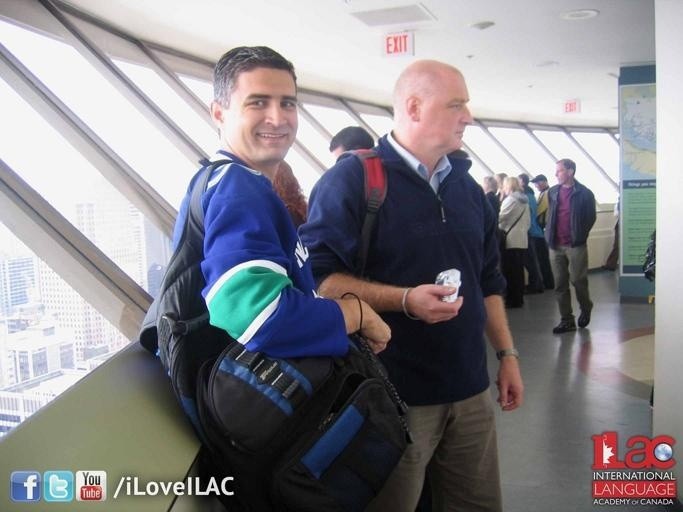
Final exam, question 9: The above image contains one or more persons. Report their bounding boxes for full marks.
[293,59,528,512]
[137,46,393,512]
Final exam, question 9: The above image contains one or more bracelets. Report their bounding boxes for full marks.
[497,347,521,361]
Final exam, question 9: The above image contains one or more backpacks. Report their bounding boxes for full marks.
[138,159,413,511]
[643,231,655,281]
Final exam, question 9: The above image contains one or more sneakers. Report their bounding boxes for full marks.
[553,322,576,333]
[578,302,593,327]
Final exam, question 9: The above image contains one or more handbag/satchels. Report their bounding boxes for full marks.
[497,230,506,250]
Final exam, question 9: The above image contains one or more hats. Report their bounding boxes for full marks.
[529,174,546,182]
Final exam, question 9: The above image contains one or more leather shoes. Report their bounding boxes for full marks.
[524,285,554,294]
[604,266,616,271]
[504,295,524,308]
